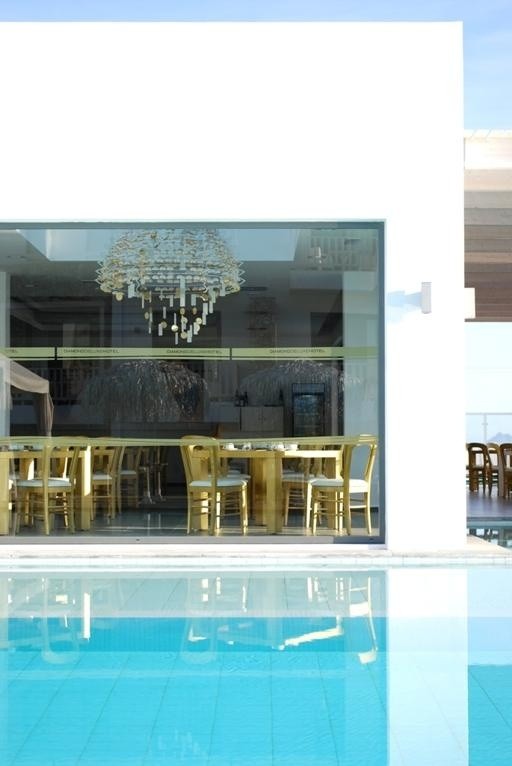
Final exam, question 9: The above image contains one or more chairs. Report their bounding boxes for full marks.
[188,577,378,666]
[466,442,512,499]
[119,446,170,510]
[180,434,378,537]
[0,435,126,536]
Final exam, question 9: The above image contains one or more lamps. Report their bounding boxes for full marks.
[93,229,246,346]
